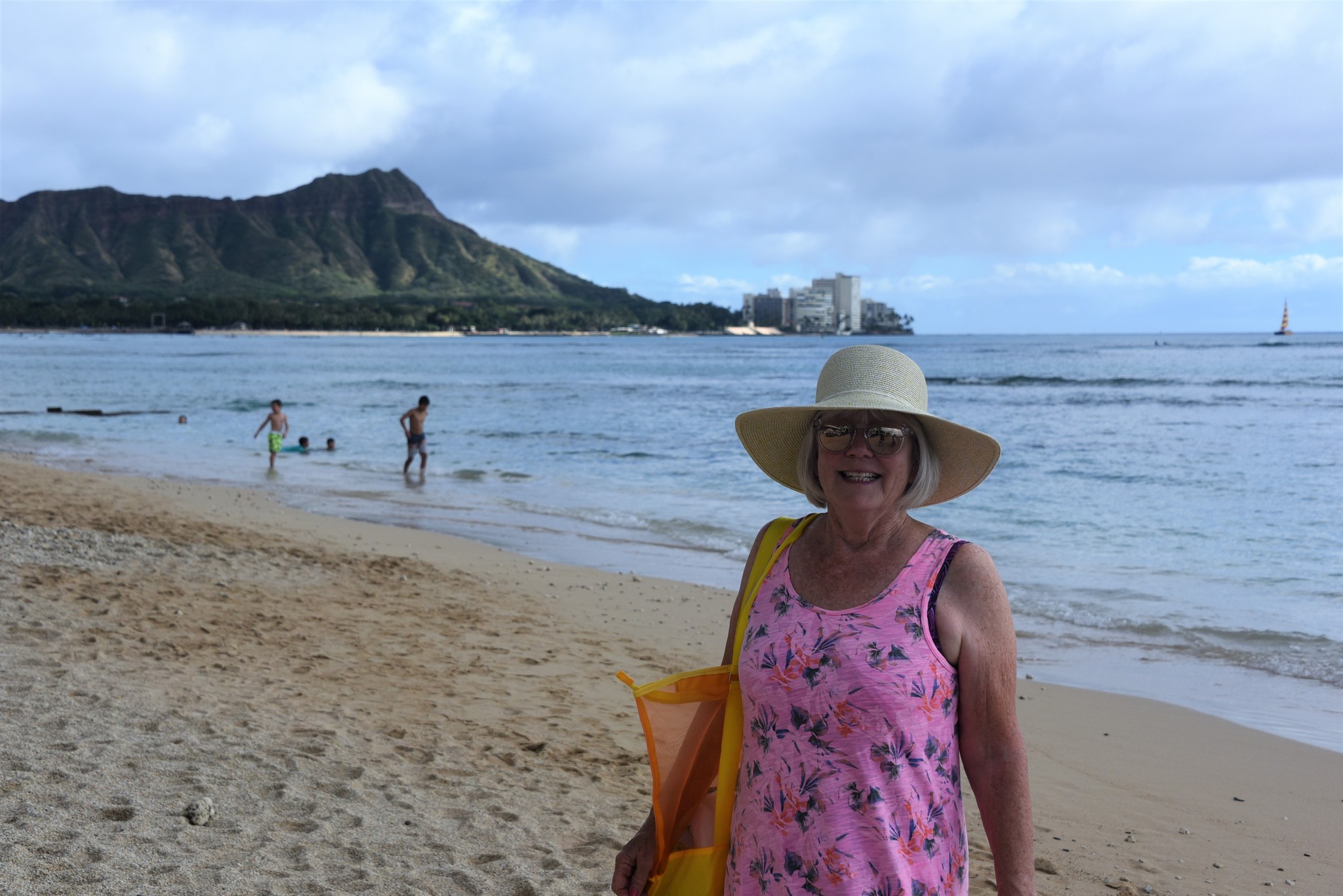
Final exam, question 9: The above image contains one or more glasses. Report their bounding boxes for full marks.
[814,421,918,458]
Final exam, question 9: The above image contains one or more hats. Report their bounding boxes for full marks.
[734,344,1001,509]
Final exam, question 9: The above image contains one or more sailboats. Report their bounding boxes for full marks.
[1274,298,1293,336]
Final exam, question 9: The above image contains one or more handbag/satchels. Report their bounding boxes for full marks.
[616,518,795,896]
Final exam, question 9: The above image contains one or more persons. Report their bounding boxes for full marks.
[253,399,289,468]
[403,473,426,495]
[325,437,337,451]
[298,436,310,453]
[609,344,1039,896]
[398,394,432,471]
[178,414,188,424]
